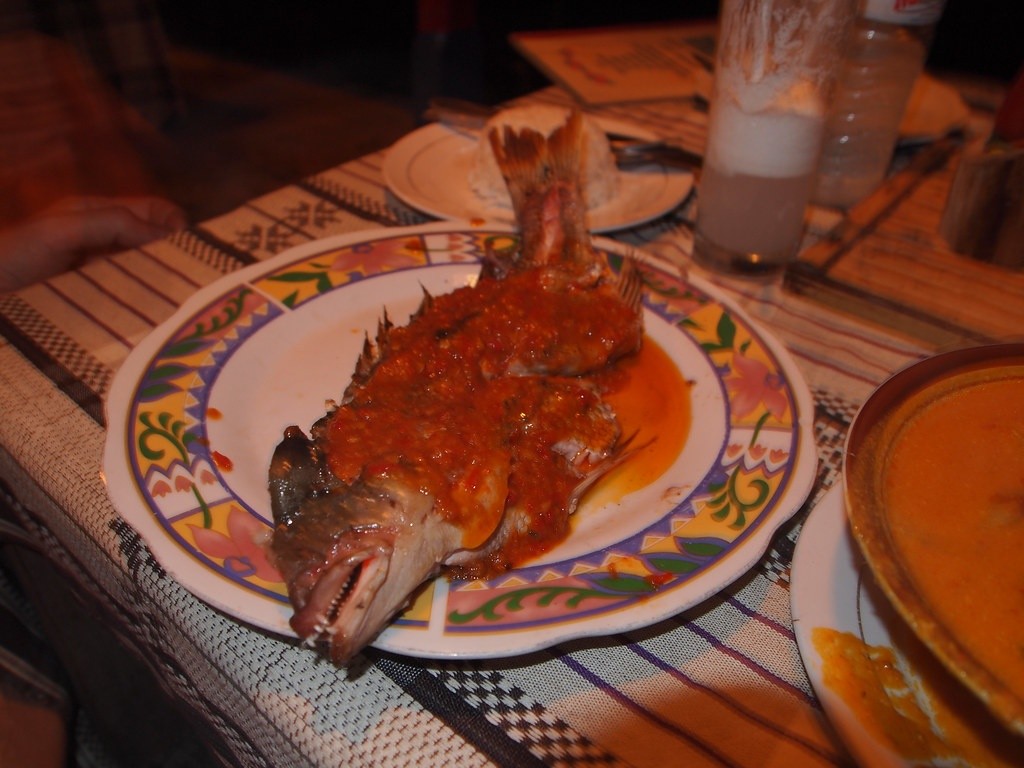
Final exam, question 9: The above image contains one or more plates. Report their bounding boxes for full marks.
[776,70,963,146]
[106,222,818,660]
[385,120,695,235]
[791,479,1024,768]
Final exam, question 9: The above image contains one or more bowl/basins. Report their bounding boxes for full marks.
[842,342,1024,743]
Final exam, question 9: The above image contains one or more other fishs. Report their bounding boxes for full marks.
[267,102,645,668]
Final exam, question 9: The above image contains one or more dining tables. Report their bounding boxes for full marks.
[0,85,1024,766]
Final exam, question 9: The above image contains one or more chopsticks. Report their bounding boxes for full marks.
[800,123,970,272]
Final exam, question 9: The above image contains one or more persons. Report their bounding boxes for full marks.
[0,193,191,768]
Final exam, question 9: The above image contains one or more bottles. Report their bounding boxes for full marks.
[804,0,941,237]
[694,0,850,286]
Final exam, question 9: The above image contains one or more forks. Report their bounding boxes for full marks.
[422,104,682,168]
